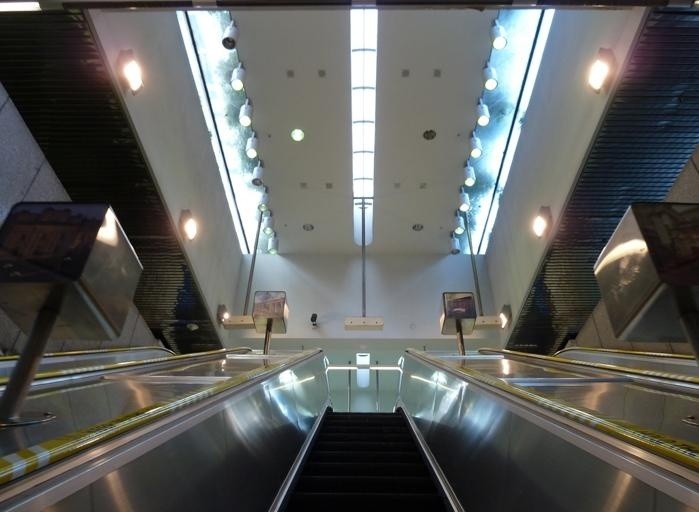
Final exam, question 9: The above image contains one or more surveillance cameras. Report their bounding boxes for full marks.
[310,313,318,326]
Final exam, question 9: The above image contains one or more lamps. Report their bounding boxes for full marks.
[110,47,147,94]
[527,206,553,239]
[492,305,514,328]
[220,18,278,256]
[450,19,507,255]
[583,47,617,92]
[176,208,198,245]
[217,304,232,327]
